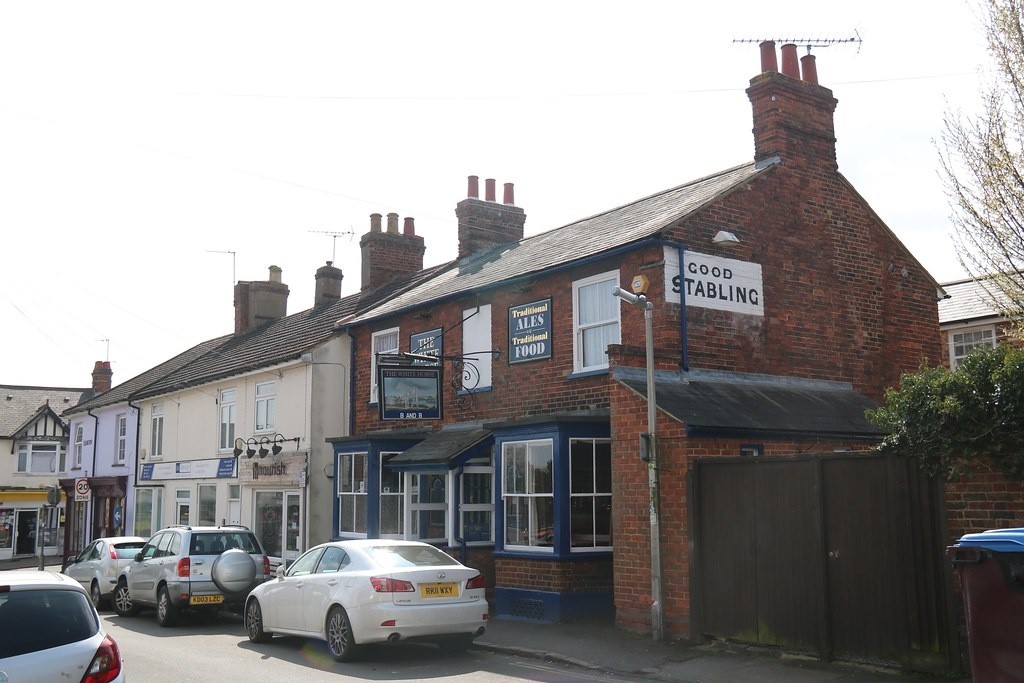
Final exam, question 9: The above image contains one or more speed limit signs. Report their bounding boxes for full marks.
[76,478,90,494]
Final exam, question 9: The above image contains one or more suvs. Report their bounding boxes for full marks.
[111,524,271,628]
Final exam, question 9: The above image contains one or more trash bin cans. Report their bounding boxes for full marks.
[946,528,1023,682]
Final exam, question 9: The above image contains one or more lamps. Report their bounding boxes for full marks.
[233,434,300,459]
[711,231,741,247]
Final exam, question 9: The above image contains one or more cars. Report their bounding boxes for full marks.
[64,536,152,610]
[244,539,489,662]
[0,570,126,683]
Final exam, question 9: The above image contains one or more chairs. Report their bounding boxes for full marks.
[194,540,224,552]
